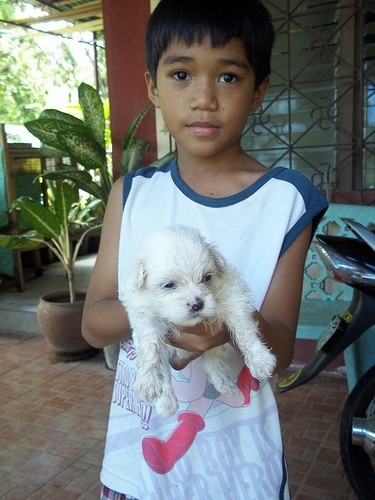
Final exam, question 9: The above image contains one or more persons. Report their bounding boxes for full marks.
[80,0,330,500]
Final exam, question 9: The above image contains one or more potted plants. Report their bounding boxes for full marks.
[0,81,177,362]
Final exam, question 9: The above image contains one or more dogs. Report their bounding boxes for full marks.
[118,224,278,418]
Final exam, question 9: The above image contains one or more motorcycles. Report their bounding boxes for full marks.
[274,216,375,500]
